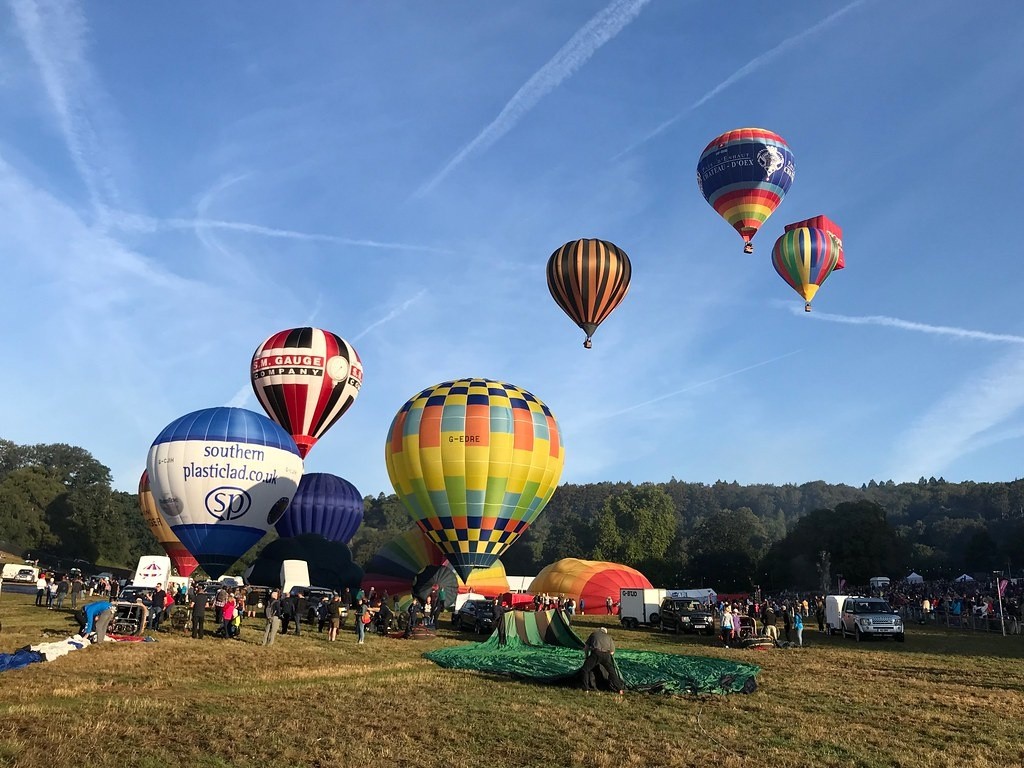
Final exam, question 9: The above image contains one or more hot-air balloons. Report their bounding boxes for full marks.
[138,468,200,576]
[771,227,840,313]
[360,527,509,621]
[695,127,798,254]
[384,377,567,599]
[146,406,304,583]
[274,472,365,547]
[524,558,654,616]
[250,327,364,463]
[784,215,845,270]
[245,532,364,591]
[545,238,633,350]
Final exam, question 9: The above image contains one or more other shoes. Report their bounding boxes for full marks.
[723,644,729,648]
[355,640,364,645]
[47,607,53,610]
[71,606,77,611]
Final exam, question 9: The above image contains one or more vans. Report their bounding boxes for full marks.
[288,586,348,626]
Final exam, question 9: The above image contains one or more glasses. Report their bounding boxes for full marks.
[156,586,159,588]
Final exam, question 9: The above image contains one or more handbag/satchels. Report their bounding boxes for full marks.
[361,611,371,623]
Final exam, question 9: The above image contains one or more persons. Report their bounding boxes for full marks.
[532,593,622,616]
[664,571,1024,648]
[36,569,446,648]
[579,626,626,694]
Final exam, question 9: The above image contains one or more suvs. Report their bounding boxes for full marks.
[841,595,906,642]
[660,596,715,637]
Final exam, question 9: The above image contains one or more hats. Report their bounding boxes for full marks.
[50,578,54,581]
[39,574,46,578]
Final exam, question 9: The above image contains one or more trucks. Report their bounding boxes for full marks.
[618,587,669,630]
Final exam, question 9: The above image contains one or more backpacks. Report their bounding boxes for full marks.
[216,590,226,602]
[265,600,280,622]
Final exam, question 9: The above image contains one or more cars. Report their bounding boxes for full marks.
[110,585,158,628]
[456,597,497,637]
[14,569,35,584]
